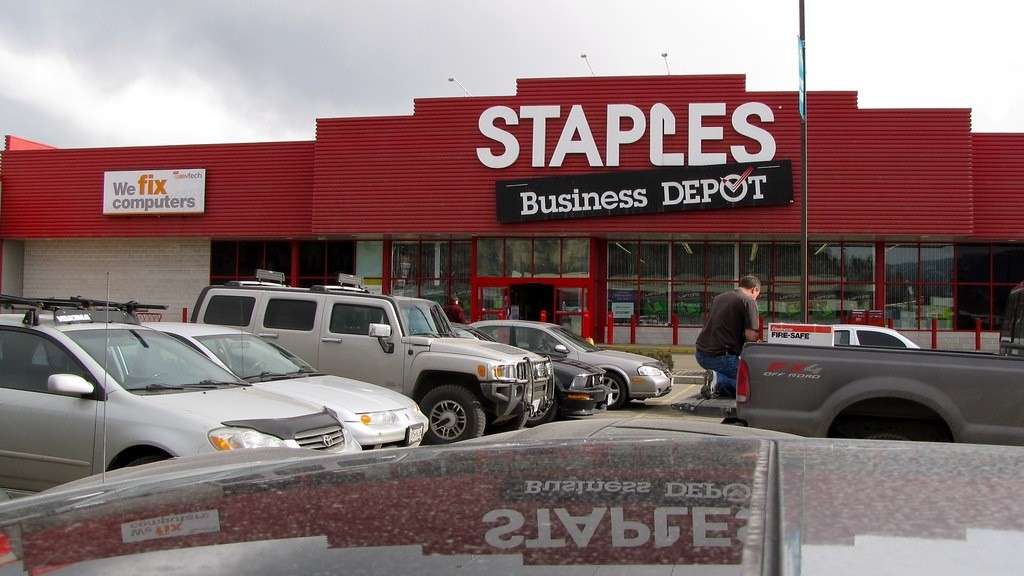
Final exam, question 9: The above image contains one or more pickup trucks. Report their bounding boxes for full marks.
[719,343,1024,447]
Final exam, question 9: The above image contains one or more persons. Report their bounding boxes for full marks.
[695,276,761,400]
[443,296,466,325]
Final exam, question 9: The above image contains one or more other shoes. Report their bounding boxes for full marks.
[701,369,717,399]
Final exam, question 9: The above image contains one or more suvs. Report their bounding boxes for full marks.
[188,270,554,445]
[1,292,365,503]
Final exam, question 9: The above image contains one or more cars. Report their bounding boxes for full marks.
[640,316,662,325]
[828,324,927,350]
[450,322,609,428]
[114,320,429,449]
[469,319,675,411]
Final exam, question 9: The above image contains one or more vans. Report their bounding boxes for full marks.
[997,281,1024,357]
